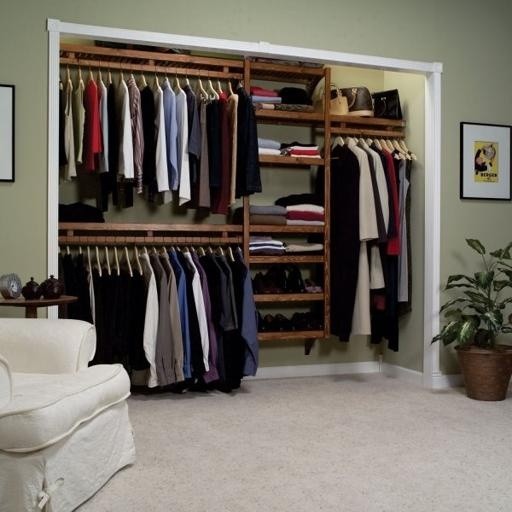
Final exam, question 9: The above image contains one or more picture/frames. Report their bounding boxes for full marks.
[460,122,512,201]
[0,83,16,183]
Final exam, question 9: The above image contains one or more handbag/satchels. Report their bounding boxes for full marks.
[315,83,403,120]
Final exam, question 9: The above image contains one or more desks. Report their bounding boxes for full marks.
[0,296,78,320]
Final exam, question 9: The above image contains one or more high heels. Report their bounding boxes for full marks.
[253,264,323,293]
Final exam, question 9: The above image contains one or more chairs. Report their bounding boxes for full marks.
[0,316,136,512]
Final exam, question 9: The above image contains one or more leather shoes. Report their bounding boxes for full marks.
[255,311,324,332]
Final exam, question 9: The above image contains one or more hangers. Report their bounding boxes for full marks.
[60,60,241,103]
[332,125,416,166]
[62,231,243,277]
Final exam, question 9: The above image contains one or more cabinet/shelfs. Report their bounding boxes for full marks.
[59,44,407,343]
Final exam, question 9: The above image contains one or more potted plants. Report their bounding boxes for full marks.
[427,239,512,404]
